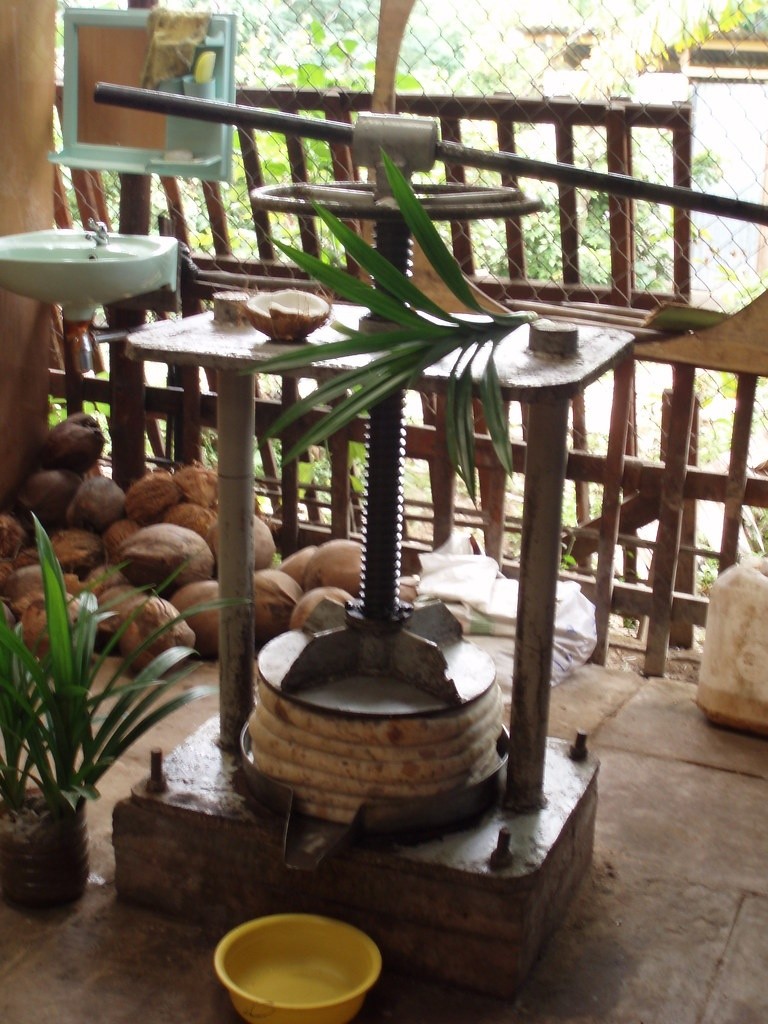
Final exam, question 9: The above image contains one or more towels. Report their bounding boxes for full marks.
[142,6,212,90]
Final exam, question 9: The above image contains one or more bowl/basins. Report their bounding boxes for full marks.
[213,914,382,1024]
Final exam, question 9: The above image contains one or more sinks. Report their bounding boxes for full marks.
[0,228,179,305]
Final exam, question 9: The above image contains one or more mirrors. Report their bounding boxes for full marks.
[74,25,163,150]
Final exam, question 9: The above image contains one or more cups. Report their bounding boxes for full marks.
[183,76,215,99]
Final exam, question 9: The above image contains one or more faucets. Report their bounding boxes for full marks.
[85,217,109,246]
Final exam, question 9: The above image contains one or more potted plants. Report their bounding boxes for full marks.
[1,504,256,908]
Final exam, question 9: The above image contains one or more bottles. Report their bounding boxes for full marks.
[694,556,768,734]
